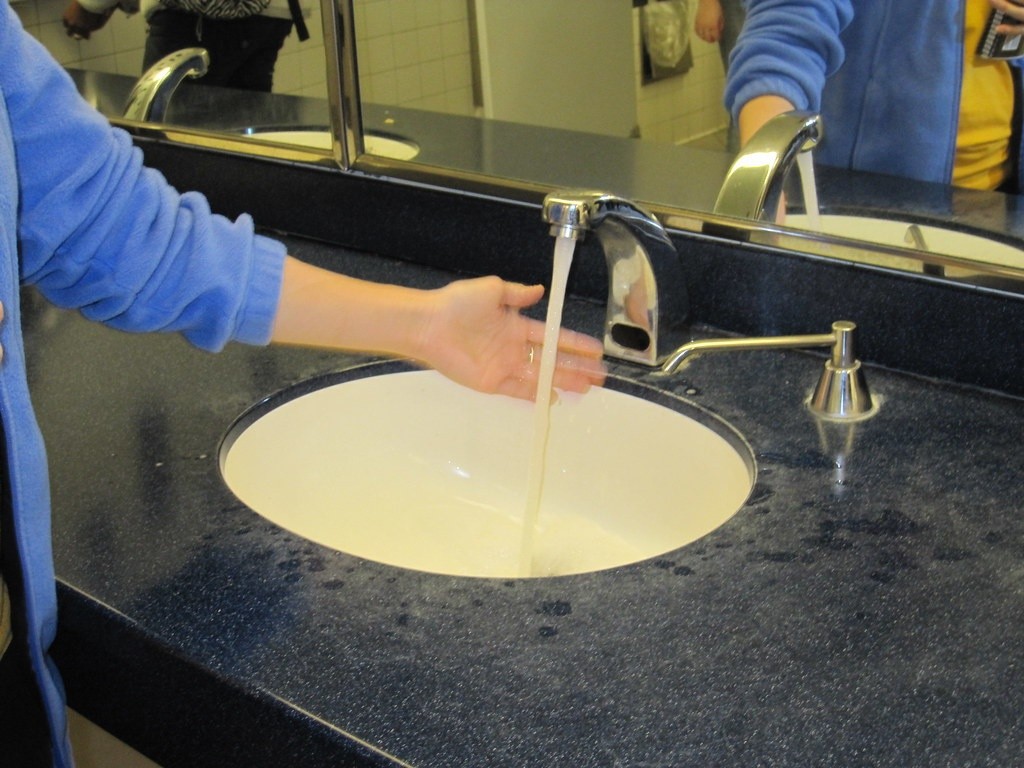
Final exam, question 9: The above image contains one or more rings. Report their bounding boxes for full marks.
[524,344,534,363]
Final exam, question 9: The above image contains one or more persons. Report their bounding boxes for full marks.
[724,0,1024,188]
[693,0,753,72]
[0,0,608,768]
[62,0,304,95]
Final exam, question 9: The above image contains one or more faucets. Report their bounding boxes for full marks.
[709,104,827,231]
[121,41,210,121]
[537,182,697,371]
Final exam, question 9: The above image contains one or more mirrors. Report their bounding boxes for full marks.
[10,0,1024,278]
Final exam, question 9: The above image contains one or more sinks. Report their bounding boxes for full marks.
[214,349,761,587]
[663,200,1024,292]
[232,116,421,171]
